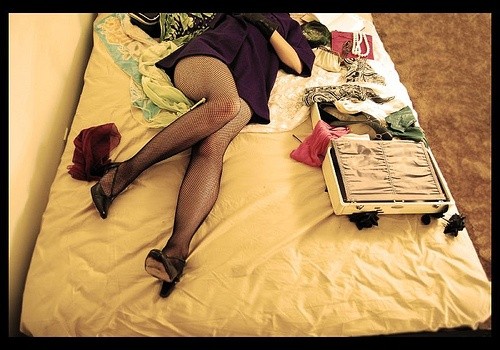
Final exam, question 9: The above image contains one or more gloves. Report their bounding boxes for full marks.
[231,13,279,40]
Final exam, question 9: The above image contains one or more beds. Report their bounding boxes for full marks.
[20,12,491,337]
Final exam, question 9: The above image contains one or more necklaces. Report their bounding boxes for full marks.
[352,33,361,55]
[355,33,370,57]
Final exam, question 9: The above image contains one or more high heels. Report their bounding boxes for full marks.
[144,249,185,298]
[91,161,118,219]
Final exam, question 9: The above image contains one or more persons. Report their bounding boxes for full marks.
[89,13,315,297]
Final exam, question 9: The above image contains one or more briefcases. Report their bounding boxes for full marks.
[310,102,455,215]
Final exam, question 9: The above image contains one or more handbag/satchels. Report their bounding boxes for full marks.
[332,30,374,59]
